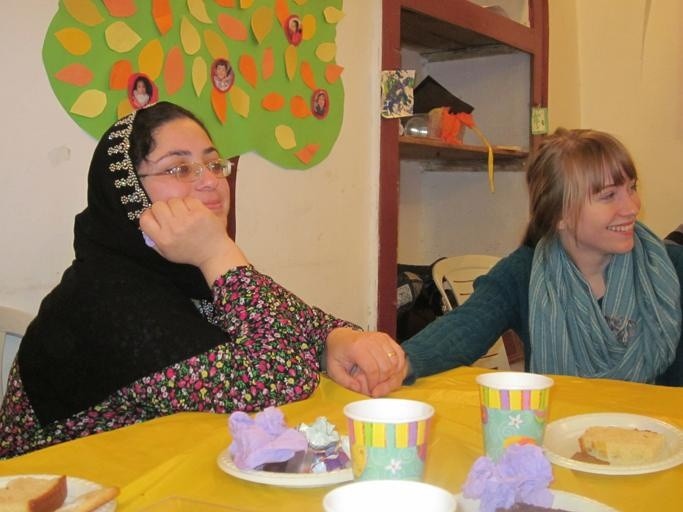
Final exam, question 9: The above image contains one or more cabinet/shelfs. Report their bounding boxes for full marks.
[377,0,549,341]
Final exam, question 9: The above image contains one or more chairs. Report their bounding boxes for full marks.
[432,254,511,371]
[0,305,36,408]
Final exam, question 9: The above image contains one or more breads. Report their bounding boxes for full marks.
[570,427,667,466]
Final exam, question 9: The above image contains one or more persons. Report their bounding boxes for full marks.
[1,101,407,462]
[288,19,302,46]
[348,121,683,399]
[131,79,150,107]
[211,59,232,92]
[312,91,325,115]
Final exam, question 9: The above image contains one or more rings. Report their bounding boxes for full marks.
[387,352,397,357]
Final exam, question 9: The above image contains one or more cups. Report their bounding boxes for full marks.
[342,398,436,489]
[475,370,555,463]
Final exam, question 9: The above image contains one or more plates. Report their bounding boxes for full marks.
[322,478,459,511]
[214,435,353,488]
[452,487,620,511]
[545,411,681,478]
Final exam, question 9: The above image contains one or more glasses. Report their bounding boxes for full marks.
[138,159,234,183]
